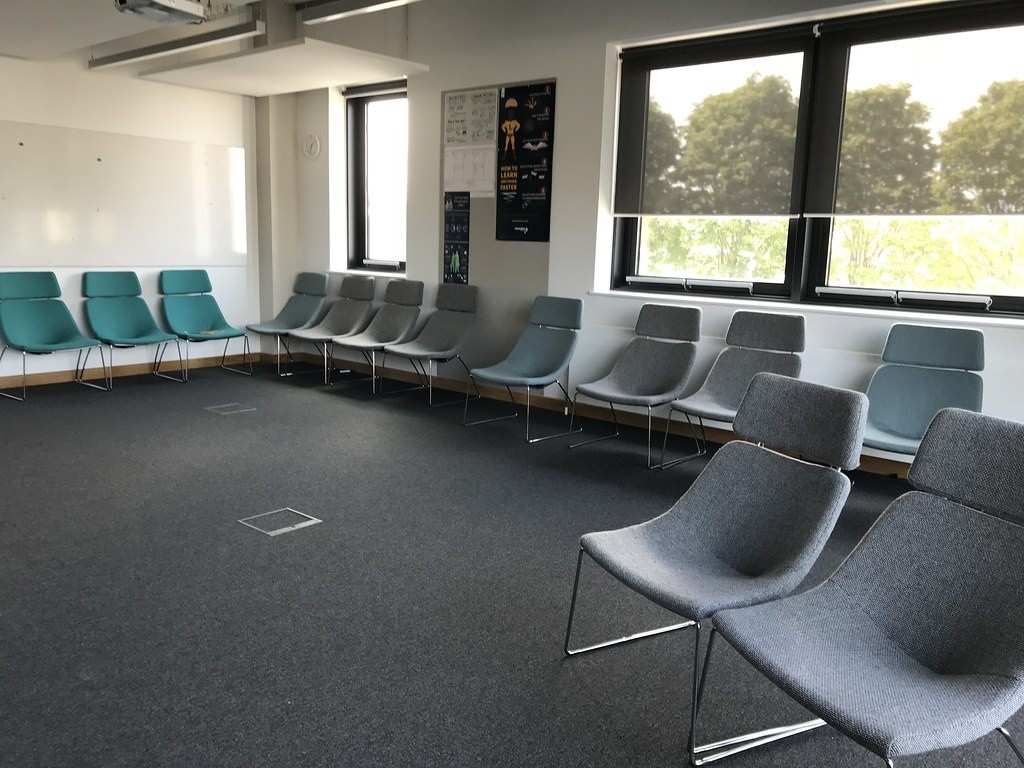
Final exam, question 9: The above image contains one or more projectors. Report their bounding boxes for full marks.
[113,0,204,26]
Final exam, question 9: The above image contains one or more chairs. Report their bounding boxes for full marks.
[243,272,328,377]
[837,324,984,487]
[566,304,701,469]
[155,269,254,382]
[74,272,186,392]
[0,271,112,403]
[376,284,483,407]
[661,312,805,466]
[689,407,1024,768]
[287,275,376,381]
[326,278,424,400]
[466,297,587,443]
[565,372,869,748]
